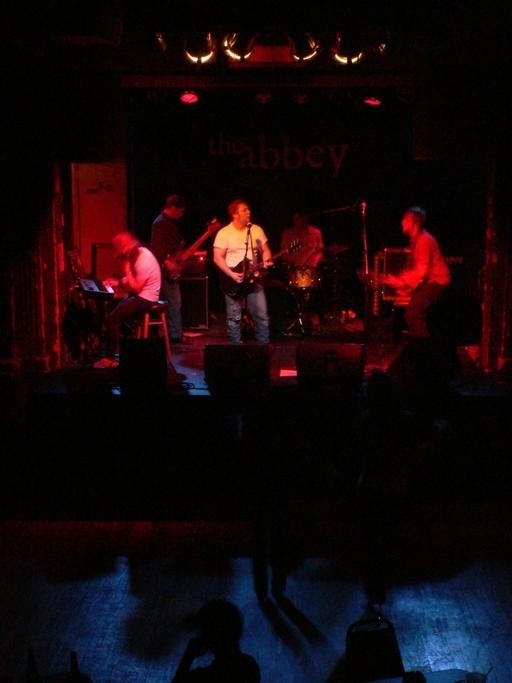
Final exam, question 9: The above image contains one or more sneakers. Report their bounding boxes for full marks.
[172,335,192,345]
[93,359,118,367]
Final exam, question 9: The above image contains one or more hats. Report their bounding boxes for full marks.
[111,233,137,258]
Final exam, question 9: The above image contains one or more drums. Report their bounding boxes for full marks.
[285,262,321,291]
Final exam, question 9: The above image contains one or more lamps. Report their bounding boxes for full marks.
[178,30,393,111]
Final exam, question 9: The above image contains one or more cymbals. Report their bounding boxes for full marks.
[319,244,349,254]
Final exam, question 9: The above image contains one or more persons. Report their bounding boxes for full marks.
[174,598,262,683]
[106,229,162,353]
[276,210,326,324]
[214,200,275,344]
[381,205,451,336]
[149,193,188,341]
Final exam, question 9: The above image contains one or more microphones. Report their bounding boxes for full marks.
[244,222,254,232]
[360,198,367,217]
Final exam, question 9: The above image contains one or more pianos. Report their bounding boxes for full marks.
[80,279,115,299]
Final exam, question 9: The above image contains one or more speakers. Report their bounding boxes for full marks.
[386,336,459,385]
[118,335,168,384]
[204,344,270,388]
[177,274,209,330]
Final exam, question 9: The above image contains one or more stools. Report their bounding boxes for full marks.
[135,295,176,375]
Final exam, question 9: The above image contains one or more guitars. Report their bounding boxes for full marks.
[222,240,305,300]
[162,218,222,283]
